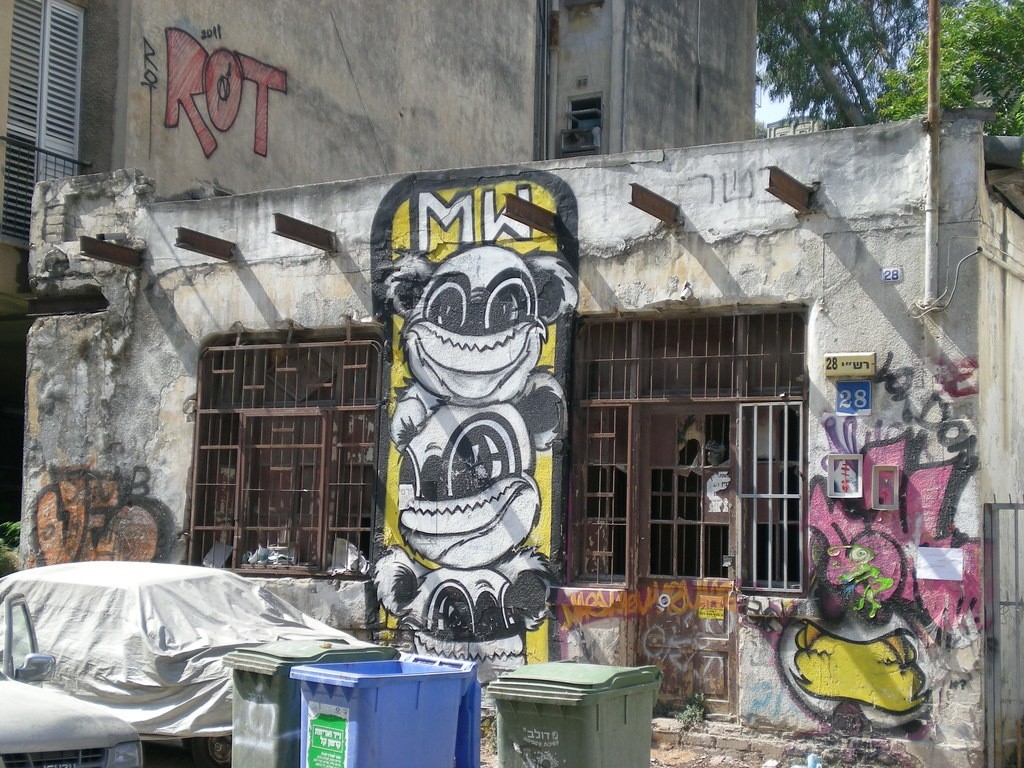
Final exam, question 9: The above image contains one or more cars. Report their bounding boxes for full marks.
[0,592,142,768]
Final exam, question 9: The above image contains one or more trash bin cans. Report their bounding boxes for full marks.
[485,659,664,768]
[222,635,401,768]
[287,652,480,768]
